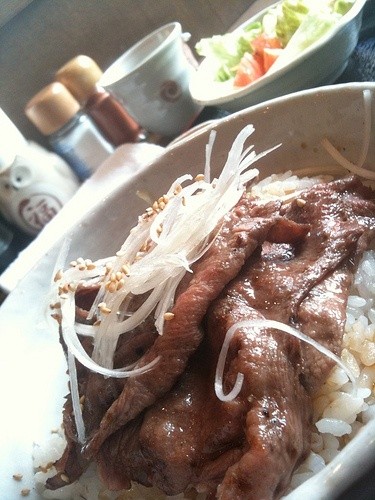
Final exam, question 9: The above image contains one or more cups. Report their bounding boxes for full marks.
[98,21,204,141]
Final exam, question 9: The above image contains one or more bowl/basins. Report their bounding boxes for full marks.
[0,80,375,499]
[189,1,367,114]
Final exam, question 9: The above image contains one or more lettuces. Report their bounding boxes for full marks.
[194,0,362,90]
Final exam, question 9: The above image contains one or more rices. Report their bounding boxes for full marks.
[32,169,374,499]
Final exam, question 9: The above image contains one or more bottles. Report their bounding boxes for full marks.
[24,81,114,180]
[0,108,82,237]
[50,55,142,148]
